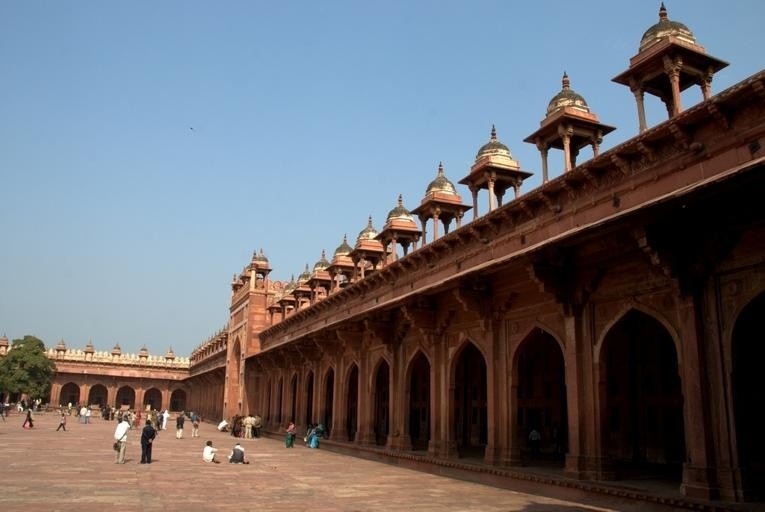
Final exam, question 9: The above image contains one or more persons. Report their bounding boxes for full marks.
[203,441,221,464]
[285,420,297,449]
[304,422,327,448]
[138,420,157,464]
[56,413,66,432]
[113,415,131,464]
[228,443,245,464]
[99,400,170,431]
[176,410,185,439]
[529,428,540,441]
[0,397,42,428]
[218,413,263,439]
[191,412,200,437]
[59,399,91,424]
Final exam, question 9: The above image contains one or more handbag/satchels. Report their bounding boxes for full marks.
[113,443,120,452]
